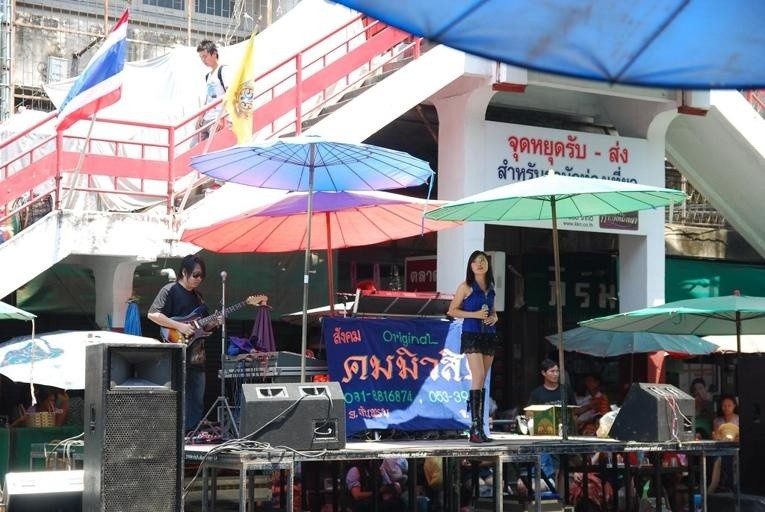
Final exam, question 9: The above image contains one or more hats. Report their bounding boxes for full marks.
[719,422,740,441]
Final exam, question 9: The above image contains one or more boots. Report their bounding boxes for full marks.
[466,389,484,443]
[480,388,493,442]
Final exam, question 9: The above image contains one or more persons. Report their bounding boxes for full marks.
[194,40,231,145]
[581,367,607,418]
[707,398,738,496]
[529,360,578,406]
[447,250,499,443]
[691,380,714,418]
[147,253,222,438]
[267,445,705,512]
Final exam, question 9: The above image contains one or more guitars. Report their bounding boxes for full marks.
[160,295,268,349]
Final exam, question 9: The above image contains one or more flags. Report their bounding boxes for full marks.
[222,35,254,147]
[54,10,128,135]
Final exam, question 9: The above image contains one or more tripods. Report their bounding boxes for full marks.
[185,284,240,446]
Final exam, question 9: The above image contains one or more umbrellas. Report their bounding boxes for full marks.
[0,301,37,323]
[576,290,765,356]
[178,190,464,318]
[545,320,720,386]
[123,290,143,336]
[248,295,276,352]
[329,0,765,90]
[0,329,163,392]
[190,134,435,383]
[423,168,690,444]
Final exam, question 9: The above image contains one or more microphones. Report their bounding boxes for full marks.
[482,304,489,323]
[221,270,228,281]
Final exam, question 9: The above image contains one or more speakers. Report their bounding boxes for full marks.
[608,382,696,443]
[80,343,187,512]
[738,352,764,495]
[238,382,347,451]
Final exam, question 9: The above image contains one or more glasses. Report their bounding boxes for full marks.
[191,270,204,279]
